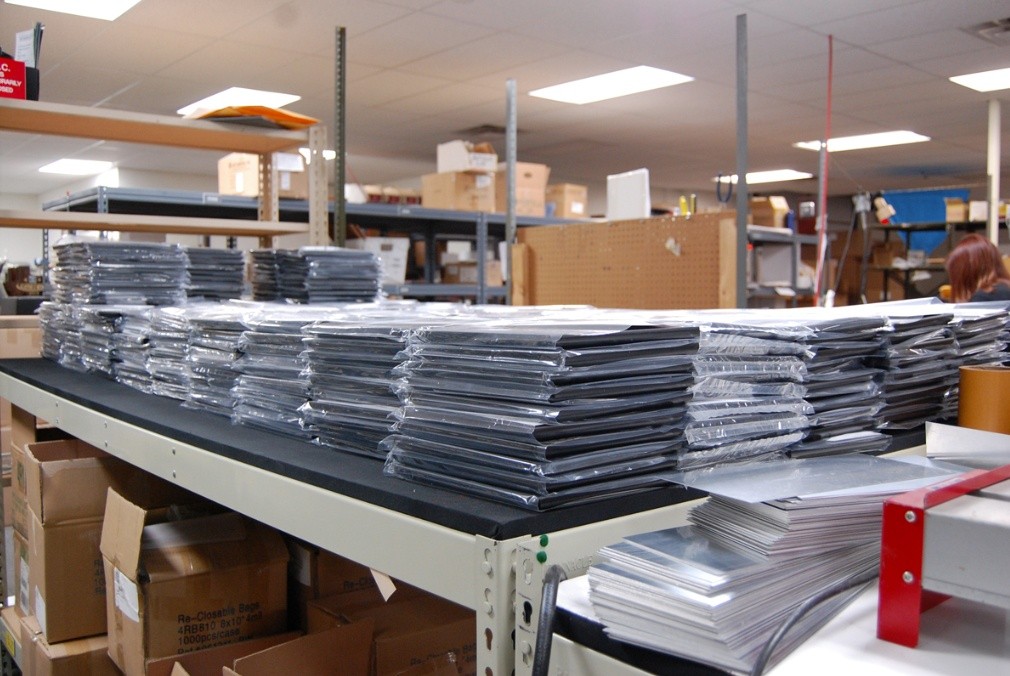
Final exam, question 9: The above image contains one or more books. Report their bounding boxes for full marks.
[589,422,1010,670]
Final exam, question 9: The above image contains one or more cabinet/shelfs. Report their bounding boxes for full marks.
[42,192,596,307]
[0,350,959,676]
[744,230,836,311]
[865,220,1010,300]
[0,100,328,329]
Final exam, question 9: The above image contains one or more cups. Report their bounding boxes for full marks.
[959,366,1010,436]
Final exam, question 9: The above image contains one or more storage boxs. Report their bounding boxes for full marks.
[945,198,968,222]
[348,238,410,284]
[421,140,588,220]
[443,257,502,288]
[0,432,479,676]
[968,200,1010,220]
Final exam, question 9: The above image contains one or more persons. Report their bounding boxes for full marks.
[944,233,1010,302]
